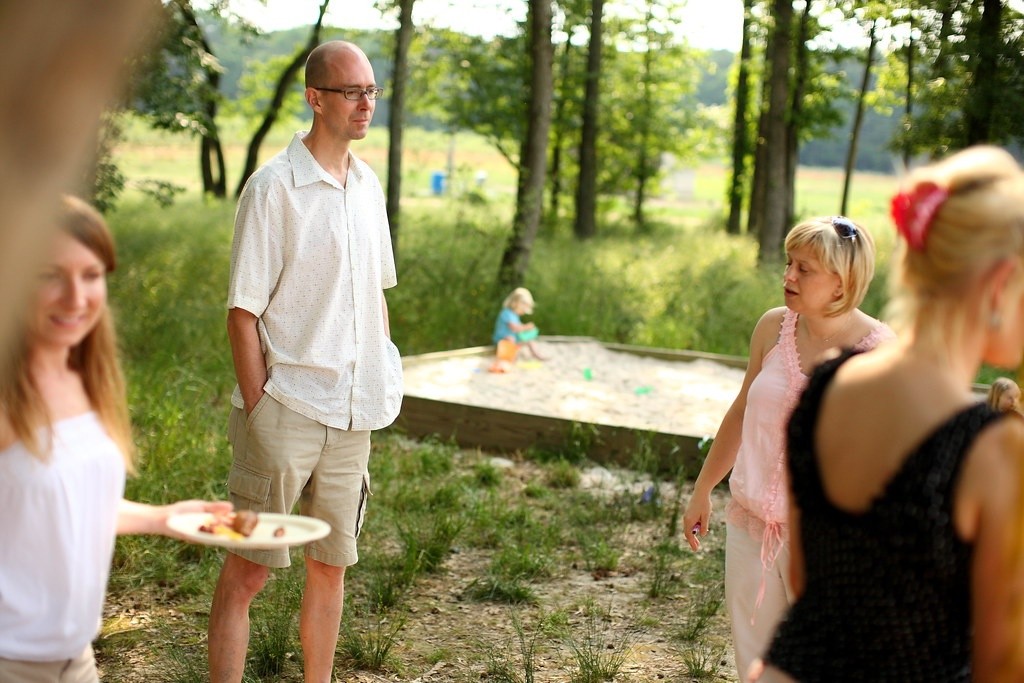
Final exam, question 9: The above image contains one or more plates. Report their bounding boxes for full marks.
[163,513,332,549]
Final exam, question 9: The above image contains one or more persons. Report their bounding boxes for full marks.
[490,287,545,361]
[681,219,895,683]
[749,146,1023,683]
[430,168,449,196]
[476,168,486,189]
[986,376,1021,416]
[0,193,235,683]
[206,42,406,683]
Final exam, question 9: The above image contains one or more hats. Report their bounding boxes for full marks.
[515,287,535,315]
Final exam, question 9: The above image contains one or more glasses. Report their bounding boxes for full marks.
[832,216,862,251]
[315,87,384,100]
